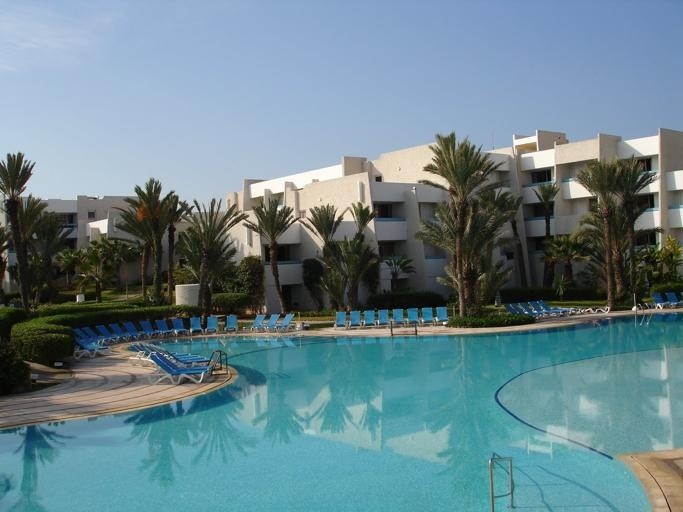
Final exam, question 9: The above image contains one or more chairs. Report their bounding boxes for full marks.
[651,292,683,309]
[504,300,575,319]
[334,307,449,331]
[73,313,296,385]
